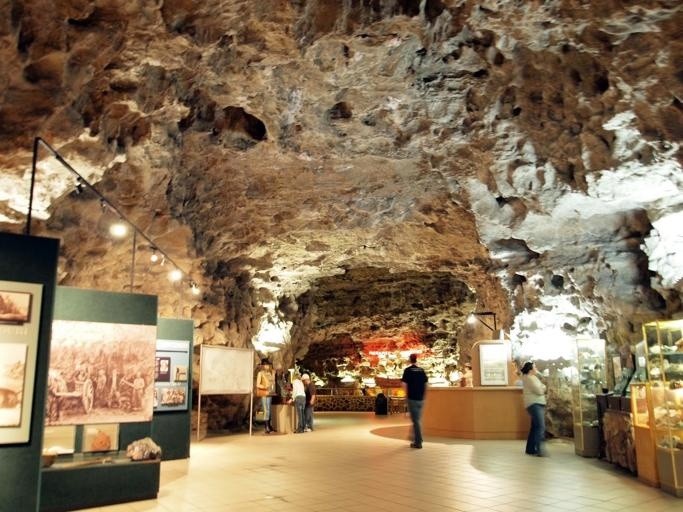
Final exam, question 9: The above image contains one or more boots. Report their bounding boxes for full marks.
[263,420,277,433]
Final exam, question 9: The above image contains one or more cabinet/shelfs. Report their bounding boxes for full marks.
[566,319,682,498]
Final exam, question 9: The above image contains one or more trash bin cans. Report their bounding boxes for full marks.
[375,393,387,415]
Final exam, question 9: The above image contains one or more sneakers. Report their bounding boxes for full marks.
[410,438,423,449]
[526,448,551,458]
[293,426,314,433]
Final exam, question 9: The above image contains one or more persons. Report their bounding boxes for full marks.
[521,362,547,456]
[401,353,428,448]
[288,373,317,432]
[256,358,275,432]
[78,365,145,410]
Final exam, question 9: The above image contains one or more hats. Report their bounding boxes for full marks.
[257,357,273,365]
[300,373,310,381]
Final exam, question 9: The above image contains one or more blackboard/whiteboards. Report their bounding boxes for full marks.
[199,344,254,395]
